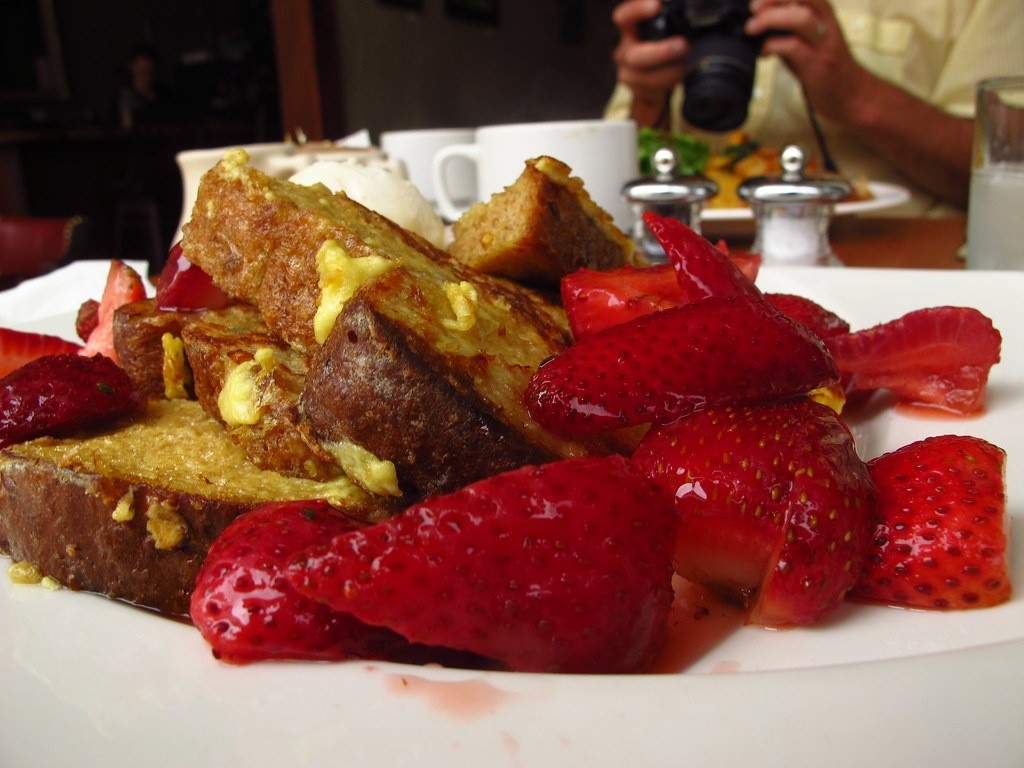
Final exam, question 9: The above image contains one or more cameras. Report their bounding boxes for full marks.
[637,1,789,132]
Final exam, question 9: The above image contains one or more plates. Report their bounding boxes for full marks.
[2,267,1024,768]
[698,179,916,234]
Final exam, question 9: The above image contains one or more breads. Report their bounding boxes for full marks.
[0,152,654,621]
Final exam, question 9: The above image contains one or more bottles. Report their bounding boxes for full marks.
[739,143,846,268]
[620,147,723,265]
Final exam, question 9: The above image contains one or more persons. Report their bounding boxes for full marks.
[604,0,1024,215]
[122,57,179,129]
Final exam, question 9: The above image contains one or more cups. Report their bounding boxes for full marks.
[175,142,441,263]
[379,128,481,223]
[436,120,641,239]
[967,75,1024,266]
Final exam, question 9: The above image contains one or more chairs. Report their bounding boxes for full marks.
[0,214,85,285]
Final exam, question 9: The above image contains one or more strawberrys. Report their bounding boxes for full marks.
[0,353,148,452]
[186,211,1011,674]
[78,256,147,369]
[0,328,87,380]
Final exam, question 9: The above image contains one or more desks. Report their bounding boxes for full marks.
[0,118,252,214]
